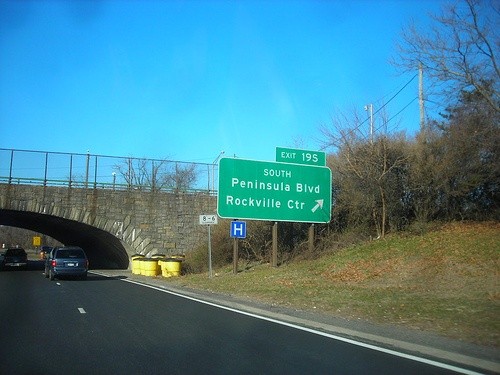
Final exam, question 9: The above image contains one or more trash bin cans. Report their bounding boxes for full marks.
[131,254,185,277]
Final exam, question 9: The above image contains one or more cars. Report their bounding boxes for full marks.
[1,247,29,270]
[41,246,89,281]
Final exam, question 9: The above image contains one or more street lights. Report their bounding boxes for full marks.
[212,150,225,195]
